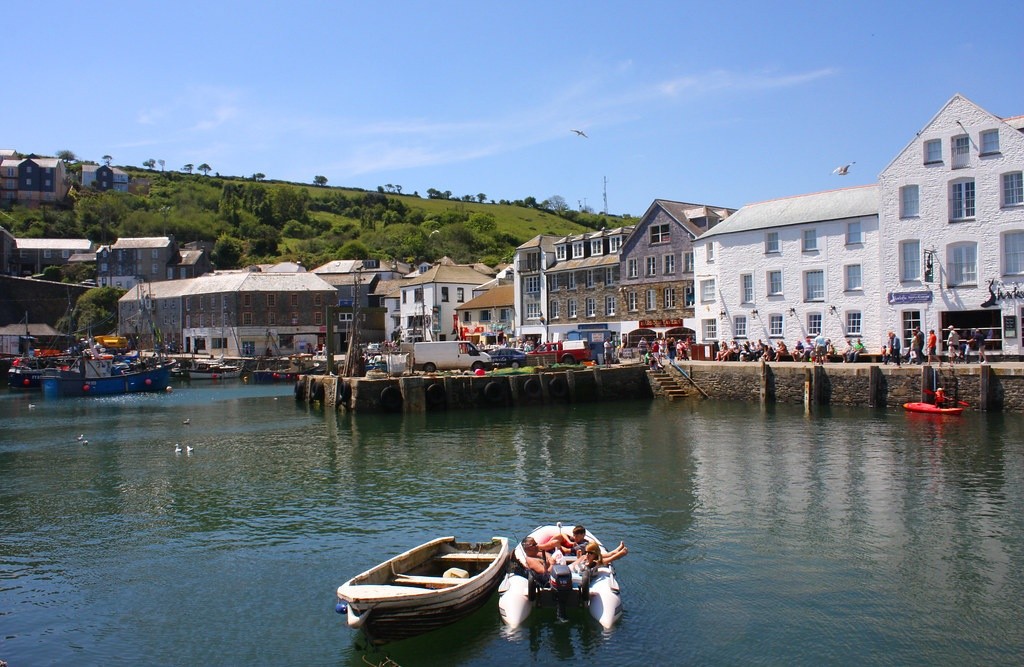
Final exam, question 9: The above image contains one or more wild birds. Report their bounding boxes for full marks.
[831,161,855,175]
[28,403,36,408]
[183,418,191,424]
[82,440,88,447]
[187,445,193,452]
[77,434,84,441]
[570,129,589,139]
[174,443,182,452]
[429,230,440,236]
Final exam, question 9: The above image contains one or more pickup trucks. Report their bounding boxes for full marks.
[528,340,593,367]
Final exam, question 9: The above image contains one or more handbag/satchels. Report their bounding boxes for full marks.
[910,349,917,359]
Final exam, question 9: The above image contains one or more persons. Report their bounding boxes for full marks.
[843,339,863,363]
[521,525,628,584]
[967,326,993,364]
[906,326,925,365]
[715,332,834,365]
[604,337,614,368]
[946,325,959,367]
[314,344,327,359]
[637,336,686,371]
[478,340,534,351]
[927,330,942,367]
[881,332,900,366]
[934,387,945,408]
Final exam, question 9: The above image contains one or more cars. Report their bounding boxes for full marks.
[363,356,388,373]
[365,343,383,356]
[488,348,529,369]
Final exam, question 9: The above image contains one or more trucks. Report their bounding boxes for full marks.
[413,340,494,372]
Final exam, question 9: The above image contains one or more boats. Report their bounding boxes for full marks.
[497,522,623,630]
[902,402,965,416]
[335,536,510,648]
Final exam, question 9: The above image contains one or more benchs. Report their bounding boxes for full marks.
[551,554,579,563]
[436,553,497,562]
[393,575,469,589]
[784,353,882,363]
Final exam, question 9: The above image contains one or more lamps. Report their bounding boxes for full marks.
[955,119,961,124]
[828,306,835,315]
[788,307,795,317]
[718,311,727,321]
[751,309,758,320]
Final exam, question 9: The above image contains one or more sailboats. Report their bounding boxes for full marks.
[4,264,365,400]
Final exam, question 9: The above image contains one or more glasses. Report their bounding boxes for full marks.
[530,543,537,548]
[585,550,596,555]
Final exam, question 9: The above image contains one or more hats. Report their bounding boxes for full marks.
[948,325,954,329]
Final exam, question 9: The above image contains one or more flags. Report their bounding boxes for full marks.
[451,315,458,334]
[924,253,933,282]
